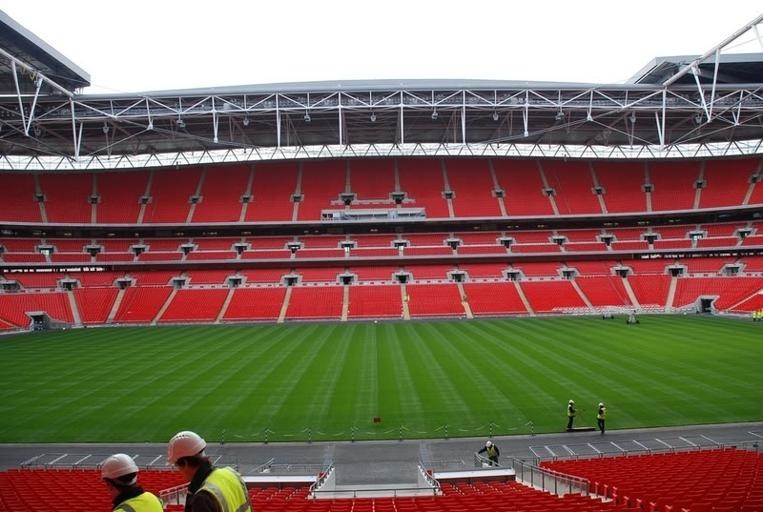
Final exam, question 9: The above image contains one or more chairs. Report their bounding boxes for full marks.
[0,448,762,512]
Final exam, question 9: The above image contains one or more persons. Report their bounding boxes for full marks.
[567,400,576,428]
[752,309,763,321]
[596,403,606,434]
[478,441,500,467]
[168,430,253,512]
[102,454,164,512]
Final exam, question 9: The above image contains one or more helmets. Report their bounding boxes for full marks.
[568,399,575,405]
[485,440,495,448]
[598,402,605,407]
[99,452,141,481]
[165,430,207,468]
